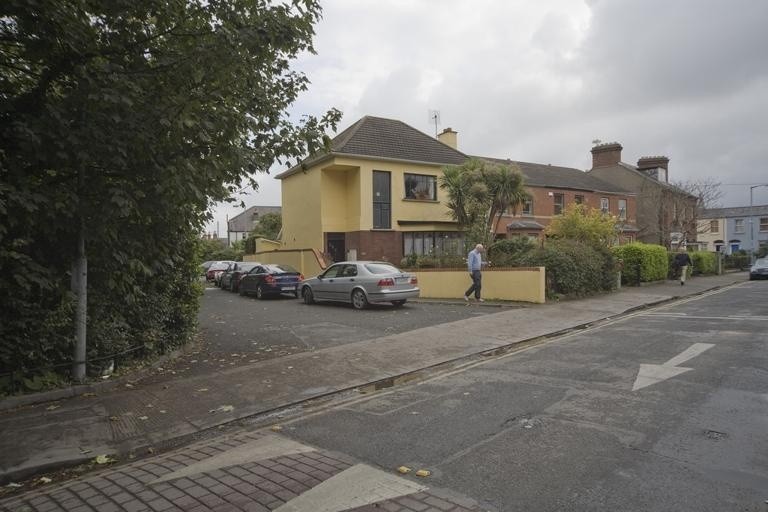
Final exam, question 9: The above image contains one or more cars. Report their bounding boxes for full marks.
[748,258,768,280]
[300,261,420,309]
[197,260,305,299]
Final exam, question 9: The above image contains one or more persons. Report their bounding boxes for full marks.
[463,244,488,303]
[675,247,693,285]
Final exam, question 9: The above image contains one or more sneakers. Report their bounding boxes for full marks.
[464,292,468,302]
[475,298,484,302]
